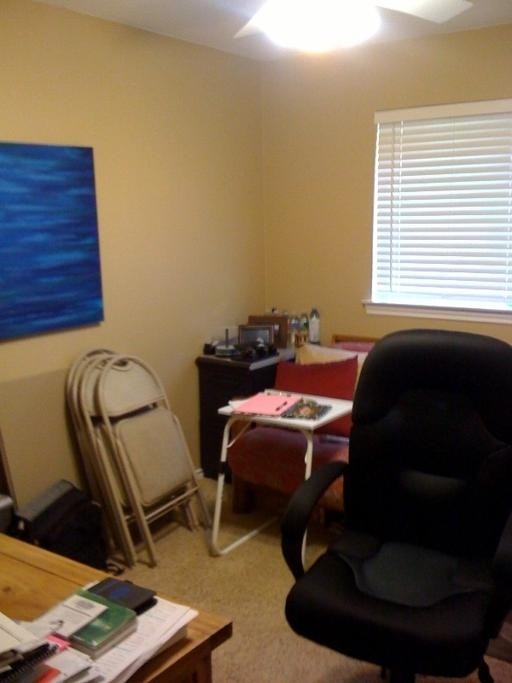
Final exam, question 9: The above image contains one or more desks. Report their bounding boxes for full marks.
[194,341,297,486]
[0,532,237,683]
[208,390,350,565]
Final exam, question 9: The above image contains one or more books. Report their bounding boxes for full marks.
[0,576,188,682]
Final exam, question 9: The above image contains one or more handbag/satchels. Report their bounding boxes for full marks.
[9,479,107,571]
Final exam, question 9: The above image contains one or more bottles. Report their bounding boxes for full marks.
[289,310,300,344]
[308,303,321,344]
[298,309,309,343]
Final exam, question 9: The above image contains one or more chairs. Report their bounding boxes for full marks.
[277,329,512,678]
[65,348,214,567]
